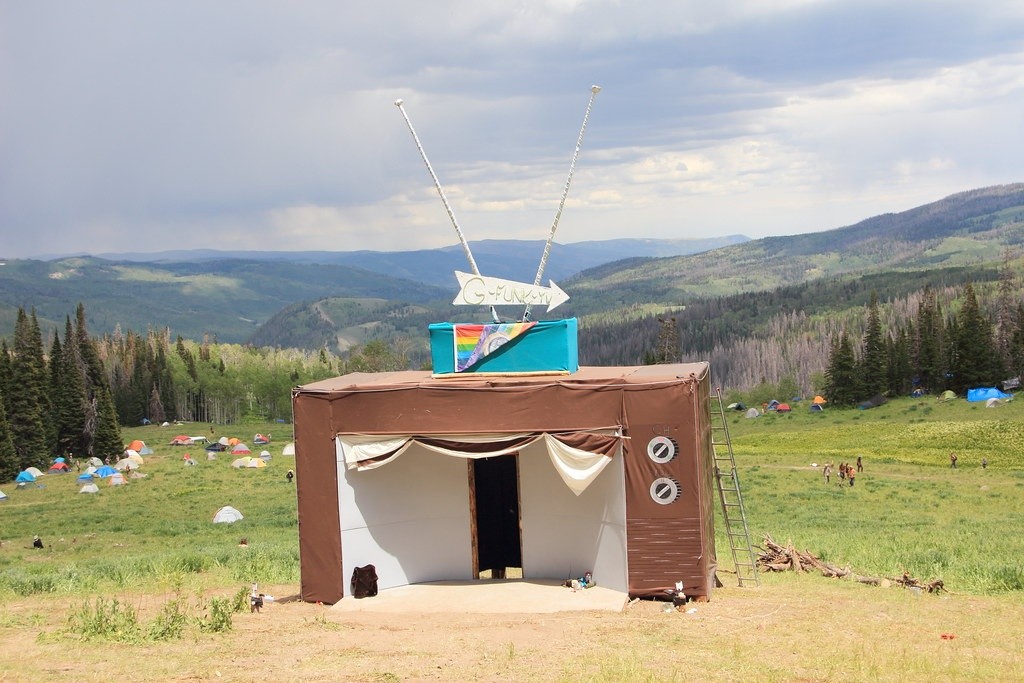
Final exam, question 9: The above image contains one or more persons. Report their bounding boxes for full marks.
[126,464,131,475]
[209,425,213,434]
[104,457,111,465]
[949,452,957,467]
[823,464,831,485]
[286,470,294,482]
[980,458,987,468]
[113,455,120,463]
[848,467,856,486]
[33,535,45,549]
[762,401,768,412]
[838,462,849,487]
[857,456,864,471]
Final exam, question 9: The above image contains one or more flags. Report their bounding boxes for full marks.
[453,321,538,371]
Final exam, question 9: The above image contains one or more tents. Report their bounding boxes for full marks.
[968,387,1012,402]
[940,389,956,402]
[0,417,296,524]
[810,404,823,411]
[986,398,1002,408]
[813,396,827,404]
[728,397,801,417]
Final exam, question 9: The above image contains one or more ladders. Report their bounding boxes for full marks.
[710,387,759,587]
